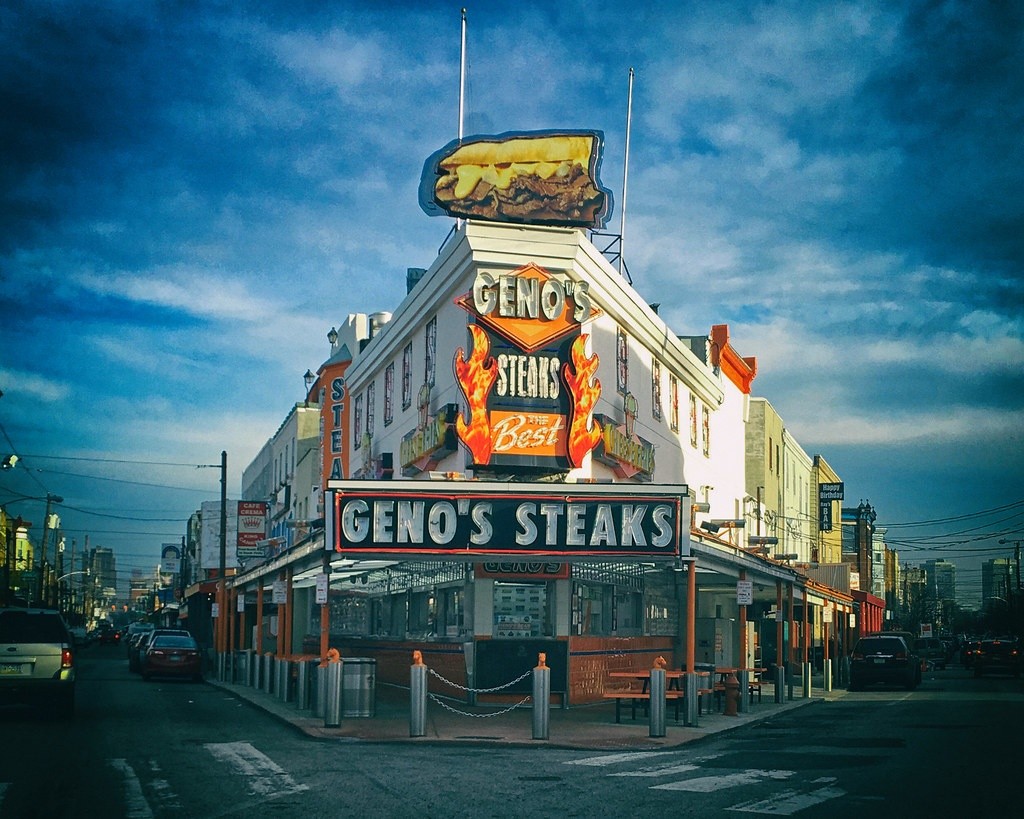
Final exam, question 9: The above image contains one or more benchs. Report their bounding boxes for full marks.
[603,682,769,723]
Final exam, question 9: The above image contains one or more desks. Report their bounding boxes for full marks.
[610,667,767,693]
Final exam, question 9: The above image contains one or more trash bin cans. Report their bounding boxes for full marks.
[342,656,377,719]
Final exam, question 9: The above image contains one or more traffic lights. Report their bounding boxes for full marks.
[124,605,128,612]
[112,605,115,611]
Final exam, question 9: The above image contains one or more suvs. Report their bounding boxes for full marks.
[0,607,75,701]
[871,632,913,653]
[849,635,922,689]
[71,630,88,648]
[99,629,120,646]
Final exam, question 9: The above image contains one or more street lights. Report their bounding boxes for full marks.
[0,495,64,507]
[986,597,1011,613]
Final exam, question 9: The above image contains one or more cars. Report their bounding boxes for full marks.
[911,638,960,669]
[958,633,1022,677]
[87,631,99,643]
[129,629,201,680]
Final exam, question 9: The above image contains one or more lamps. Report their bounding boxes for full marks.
[303,369,315,392]
[327,326,338,348]
